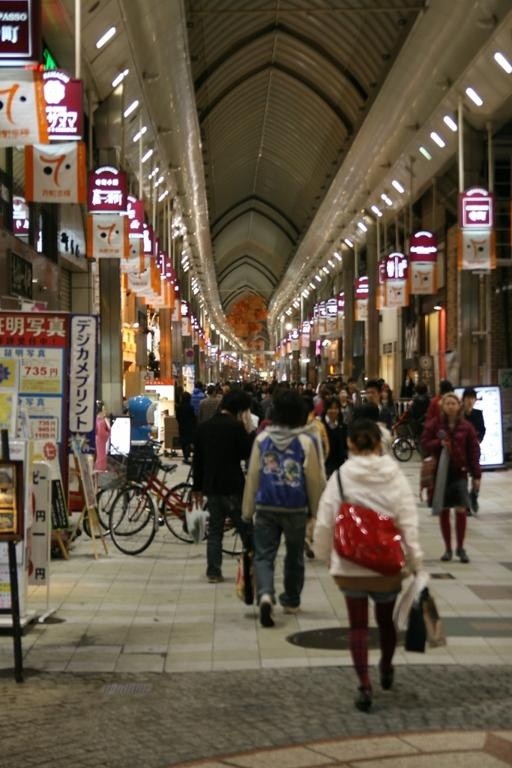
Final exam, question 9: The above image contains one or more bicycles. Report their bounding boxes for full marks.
[81,439,250,559]
[391,419,417,462]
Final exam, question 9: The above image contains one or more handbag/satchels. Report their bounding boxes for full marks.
[404,602,426,654]
[235,549,256,606]
[334,469,405,578]
[422,589,447,649]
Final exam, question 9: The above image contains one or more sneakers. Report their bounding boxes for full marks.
[283,606,299,615]
[354,694,371,710]
[455,548,469,562]
[208,576,224,583]
[259,593,275,627]
[380,664,395,691]
[440,550,452,561]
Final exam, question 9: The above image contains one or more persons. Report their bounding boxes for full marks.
[192,390,256,585]
[320,397,348,478]
[223,376,358,398]
[197,385,221,426]
[355,381,392,430]
[426,380,454,420]
[313,420,422,702]
[421,393,481,564]
[191,382,206,405]
[303,406,329,558]
[337,389,354,426]
[460,389,486,515]
[176,391,195,463]
[407,382,429,433]
[239,395,321,627]
